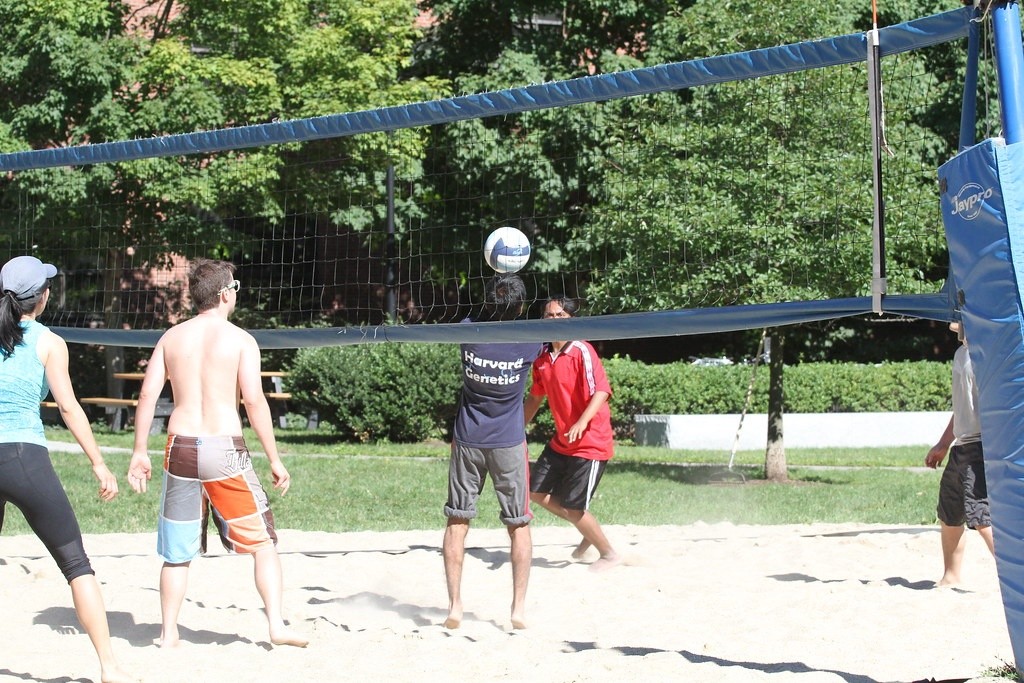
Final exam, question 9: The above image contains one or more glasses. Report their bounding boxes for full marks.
[216,279,240,296]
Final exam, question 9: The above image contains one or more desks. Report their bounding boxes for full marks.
[113,371,292,430]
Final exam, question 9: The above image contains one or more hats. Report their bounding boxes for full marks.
[0,255,59,301]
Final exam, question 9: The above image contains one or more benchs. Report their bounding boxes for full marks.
[241,392,317,431]
[79,396,138,433]
[41,401,58,408]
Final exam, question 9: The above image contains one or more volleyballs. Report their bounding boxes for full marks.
[484,226,533,275]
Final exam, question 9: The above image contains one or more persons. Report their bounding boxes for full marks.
[0,256,140,683]
[127,260,310,649]
[443,275,546,630]
[925,325,995,586]
[523,294,618,569]
